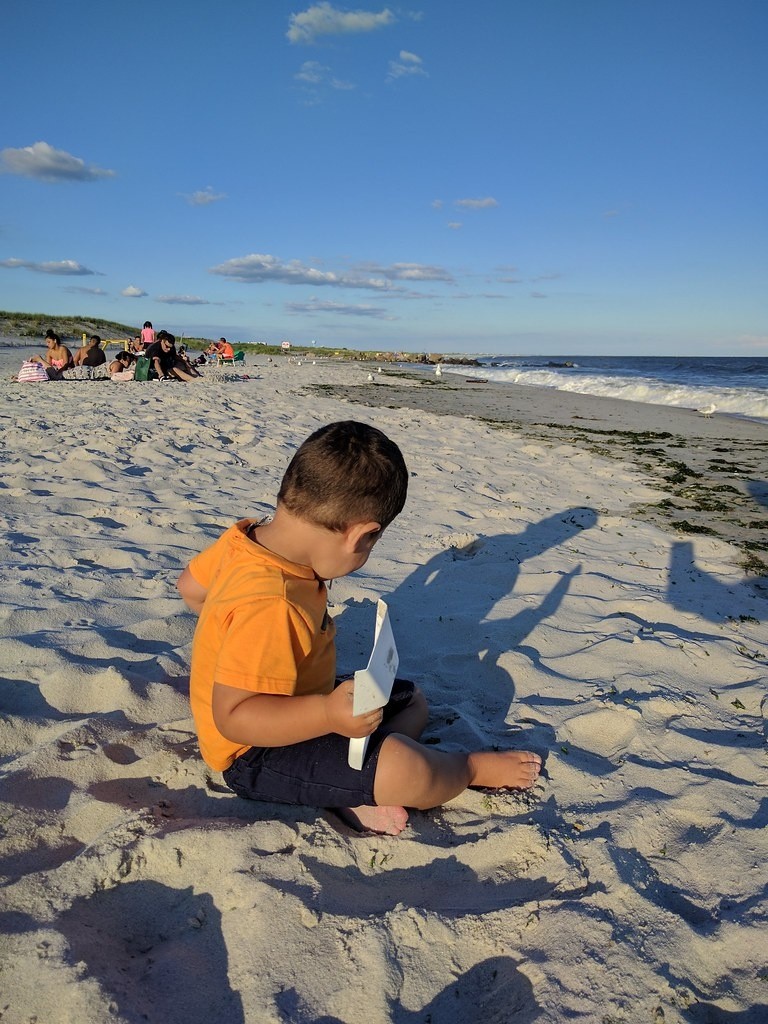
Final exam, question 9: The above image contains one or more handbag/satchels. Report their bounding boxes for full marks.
[17,357,51,382]
[135,355,151,381]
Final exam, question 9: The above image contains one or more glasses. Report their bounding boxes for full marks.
[164,342,172,349]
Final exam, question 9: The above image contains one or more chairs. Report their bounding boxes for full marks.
[100,340,128,351]
[217,351,245,367]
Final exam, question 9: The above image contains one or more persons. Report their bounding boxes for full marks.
[177,420,543,837]
[29,321,234,383]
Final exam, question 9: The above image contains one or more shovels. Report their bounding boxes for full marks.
[346,597,400,772]
